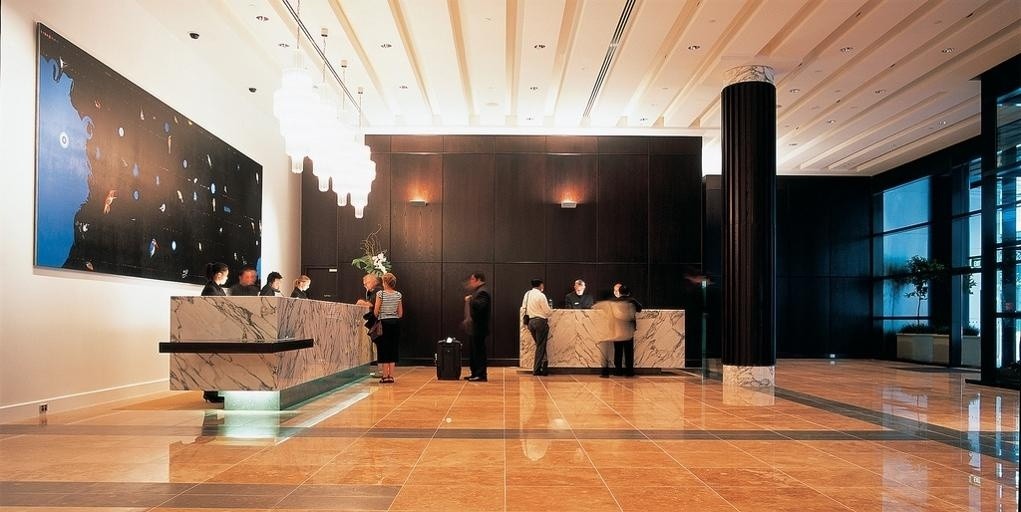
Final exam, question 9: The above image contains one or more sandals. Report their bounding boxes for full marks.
[388,376,393,382]
[378,376,388,383]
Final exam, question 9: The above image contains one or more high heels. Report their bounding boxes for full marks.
[203,392,224,403]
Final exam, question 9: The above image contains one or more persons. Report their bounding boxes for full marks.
[201,262,226,403]
[290,274,312,299]
[564,278,594,310]
[604,281,624,305]
[522,278,551,376]
[225,265,259,296]
[612,285,642,375]
[462,271,491,382]
[373,272,403,384]
[355,273,382,365]
[259,272,286,297]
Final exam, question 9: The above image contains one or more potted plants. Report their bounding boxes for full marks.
[962,272,981,368]
[895,254,950,364]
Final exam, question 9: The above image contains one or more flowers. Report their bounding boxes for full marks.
[351,253,392,278]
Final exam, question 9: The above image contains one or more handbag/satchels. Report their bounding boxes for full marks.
[522,292,530,325]
[367,290,383,342]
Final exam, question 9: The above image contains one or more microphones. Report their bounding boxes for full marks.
[272,287,280,293]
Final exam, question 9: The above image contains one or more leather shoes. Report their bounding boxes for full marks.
[464,375,475,380]
[542,360,548,376]
[468,376,487,381]
[533,371,542,376]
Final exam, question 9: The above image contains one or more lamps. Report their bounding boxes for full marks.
[273,0,377,219]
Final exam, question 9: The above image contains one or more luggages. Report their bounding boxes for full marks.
[435,336,463,380]
